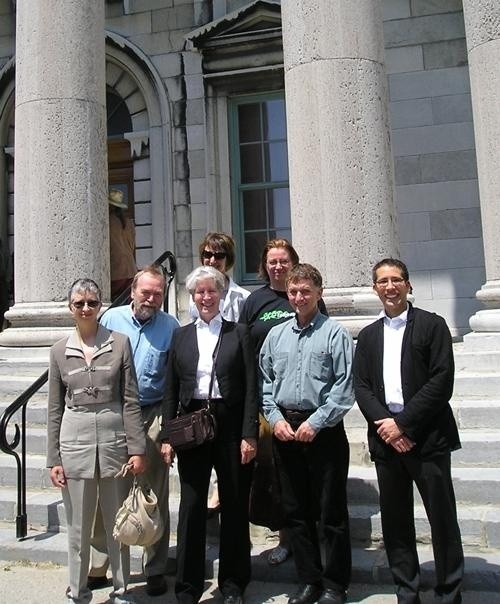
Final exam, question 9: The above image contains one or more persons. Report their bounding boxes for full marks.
[64,267,182,598]
[352,258,465,604]
[238,238,330,566]
[186,232,251,515]
[258,262,357,604]
[108,186,139,304]
[46,278,147,604]
[157,266,261,603]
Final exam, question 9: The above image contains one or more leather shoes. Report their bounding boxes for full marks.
[222,593,243,604]
[268,544,289,566]
[315,588,348,604]
[146,575,166,596]
[288,585,322,604]
[87,575,106,588]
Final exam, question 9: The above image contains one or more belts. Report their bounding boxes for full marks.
[285,409,310,420]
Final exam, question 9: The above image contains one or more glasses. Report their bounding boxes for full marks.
[71,299,98,308]
[202,249,226,260]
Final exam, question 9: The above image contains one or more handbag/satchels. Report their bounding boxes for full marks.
[164,409,217,453]
[112,486,164,545]
